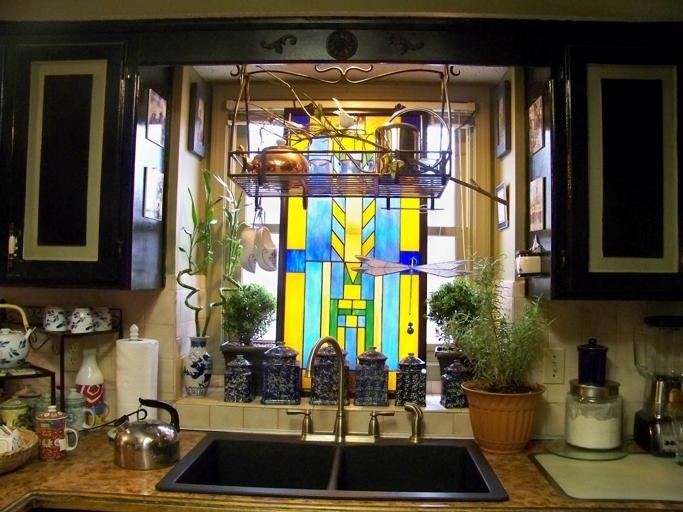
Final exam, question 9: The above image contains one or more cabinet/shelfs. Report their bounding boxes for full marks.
[522,47,681,296]
[0,38,166,288]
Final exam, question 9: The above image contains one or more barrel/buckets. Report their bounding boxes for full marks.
[373,122,418,182]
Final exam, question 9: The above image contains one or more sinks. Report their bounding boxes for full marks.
[158,431,335,498]
[335,440,492,500]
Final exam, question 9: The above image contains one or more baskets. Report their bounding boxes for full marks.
[0,424,40,475]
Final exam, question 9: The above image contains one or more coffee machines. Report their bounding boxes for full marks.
[632,314,683,456]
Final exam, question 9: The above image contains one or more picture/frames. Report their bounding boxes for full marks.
[184,79,210,157]
[496,178,509,231]
[494,78,513,160]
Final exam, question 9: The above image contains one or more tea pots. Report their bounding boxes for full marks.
[0,328,33,369]
[236,140,309,188]
[111,397,182,470]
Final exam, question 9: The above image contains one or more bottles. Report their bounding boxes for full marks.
[577,338,607,386]
[224,341,467,407]
[516,234,543,273]
[563,378,624,450]
[308,160,376,195]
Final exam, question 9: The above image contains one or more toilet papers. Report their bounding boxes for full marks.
[116,338,158,426]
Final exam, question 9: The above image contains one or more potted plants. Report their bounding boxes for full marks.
[219,284,285,395]
[174,172,248,398]
[426,278,478,412]
[439,258,558,456]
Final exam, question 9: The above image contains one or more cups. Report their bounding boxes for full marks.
[0,383,62,429]
[64,388,96,433]
[670,407,683,464]
[35,405,78,461]
[41,304,112,334]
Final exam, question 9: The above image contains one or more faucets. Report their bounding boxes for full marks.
[405,402,423,437]
[305,337,346,435]
